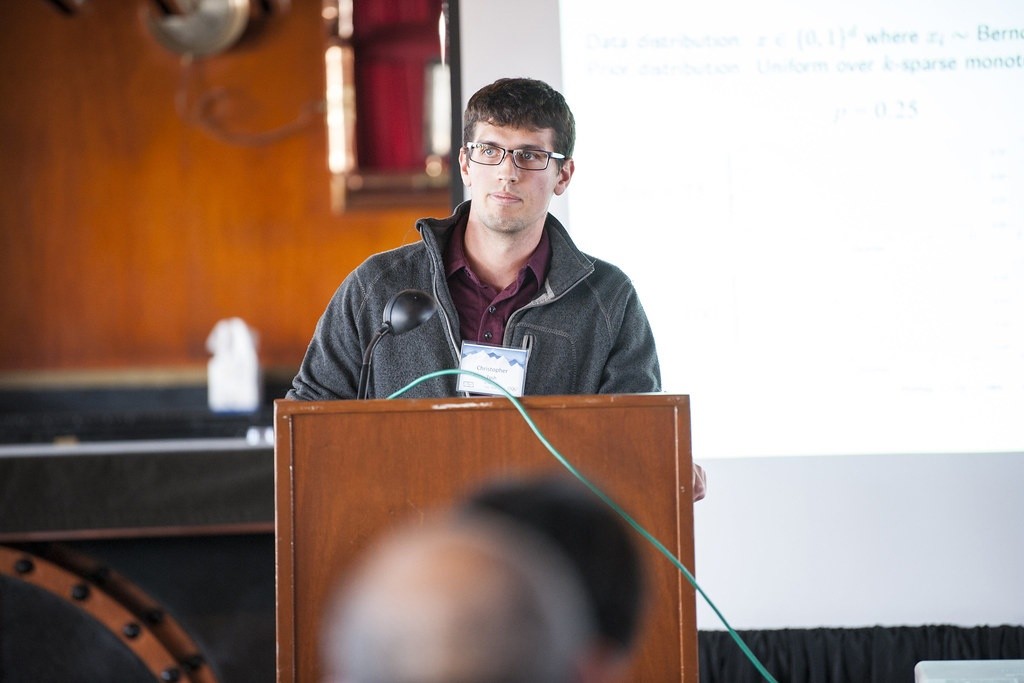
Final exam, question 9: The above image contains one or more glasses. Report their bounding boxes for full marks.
[467,141,565,171]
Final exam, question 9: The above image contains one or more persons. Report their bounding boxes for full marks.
[286,77,706,507]
[320,477,643,682]
